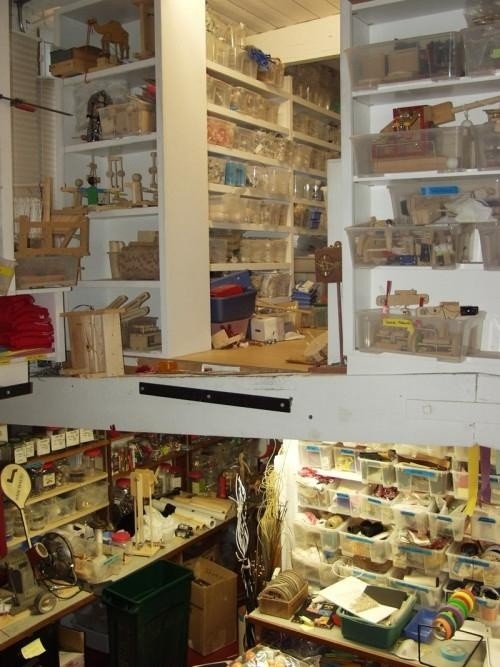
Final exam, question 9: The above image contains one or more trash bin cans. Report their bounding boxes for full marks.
[100,558,193,667]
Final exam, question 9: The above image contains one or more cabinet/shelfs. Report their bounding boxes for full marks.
[335,3,500,383]
[41,3,209,360]
[1,432,192,558]
[292,439,499,622]
[206,56,338,327]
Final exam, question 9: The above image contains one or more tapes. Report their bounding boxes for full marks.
[439,643,466,662]
[432,588,475,641]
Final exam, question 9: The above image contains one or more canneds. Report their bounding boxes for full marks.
[0,426,95,470]
[157,463,205,497]
[37,464,56,496]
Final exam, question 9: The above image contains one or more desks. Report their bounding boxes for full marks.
[1,502,254,647]
[245,601,493,667]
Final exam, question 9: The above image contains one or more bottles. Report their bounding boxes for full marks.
[1,428,205,565]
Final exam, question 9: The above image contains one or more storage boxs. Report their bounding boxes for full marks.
[55,555,239,666]
